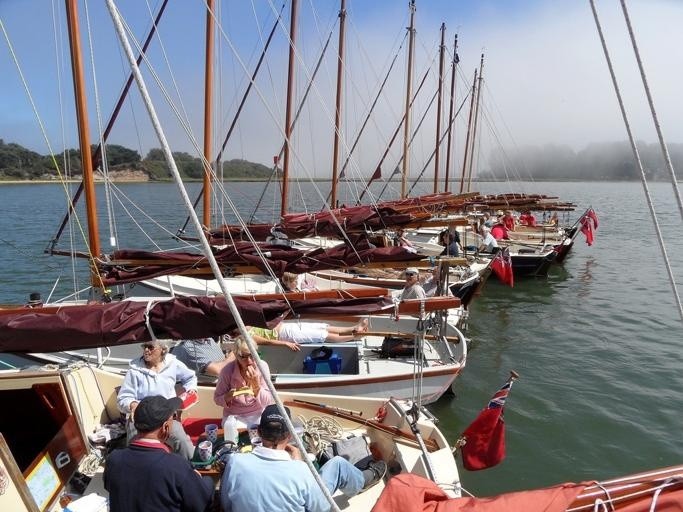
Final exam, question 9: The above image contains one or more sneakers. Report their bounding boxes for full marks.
[357,460,387,495]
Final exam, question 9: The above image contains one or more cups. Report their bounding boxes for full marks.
[248,425,263,447]
[205,424,218,444]
[198,441,212,461]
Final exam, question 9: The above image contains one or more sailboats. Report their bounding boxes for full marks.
[0,0,683,512]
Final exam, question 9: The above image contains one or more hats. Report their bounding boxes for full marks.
[405,267,419,274]
[260,404,290,442]
[134,396,181,433]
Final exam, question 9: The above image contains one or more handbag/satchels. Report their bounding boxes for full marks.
[379,336,418,359]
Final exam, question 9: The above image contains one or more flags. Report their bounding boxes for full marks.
[489,248,514,287]
[458,375,514,472]
[580,210,598,246]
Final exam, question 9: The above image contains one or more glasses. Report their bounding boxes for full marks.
[144,344,154,350]
[406,272,413,276]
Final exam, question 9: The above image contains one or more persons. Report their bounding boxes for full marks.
[440,230,463,251]
[218,404,388,512]
[171,337,236,384]
[116,340,200,459]
[438,231,464,257]
[281,272,302,294]
[213,338,273,447]
[395,228,412,247]
[102,394,215,512]
[391,267,425,304]
[230,318,368,352]
[477,209,536,253]
